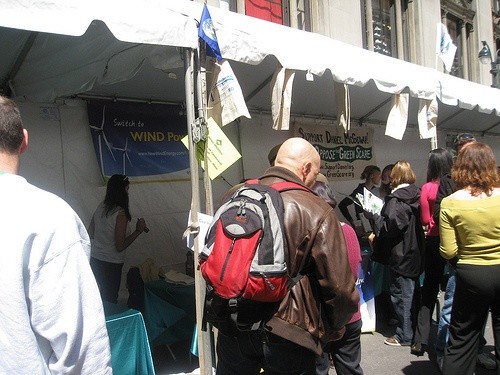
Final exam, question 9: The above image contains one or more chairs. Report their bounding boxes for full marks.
[126,266,188,361]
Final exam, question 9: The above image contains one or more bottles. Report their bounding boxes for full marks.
[185,250,194,277]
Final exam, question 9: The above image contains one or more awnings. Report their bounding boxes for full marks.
[0,1,500,130]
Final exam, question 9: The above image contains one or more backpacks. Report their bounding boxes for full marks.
[199,177,314,304]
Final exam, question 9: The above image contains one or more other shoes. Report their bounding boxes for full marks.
[412,342,425,355]
[437,355,444,372]
[476,352,496,369]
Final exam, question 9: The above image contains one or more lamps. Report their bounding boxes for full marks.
[478,41,500,87]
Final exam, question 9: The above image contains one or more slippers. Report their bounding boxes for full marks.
[384,336,413,346]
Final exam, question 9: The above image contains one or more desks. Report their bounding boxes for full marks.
[103,299,156,375]
[146,280,194,305]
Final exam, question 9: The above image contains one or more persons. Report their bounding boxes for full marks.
[378,163,393,205]
[87,174,146,302]
[0,95,112,375]
[439,142,500,375]
[412,148,460,354]
[213,136,361,375]
[304,171,363,375]
[338,165,381,230]
[367,160,422,346]
[432,132,487,356]
